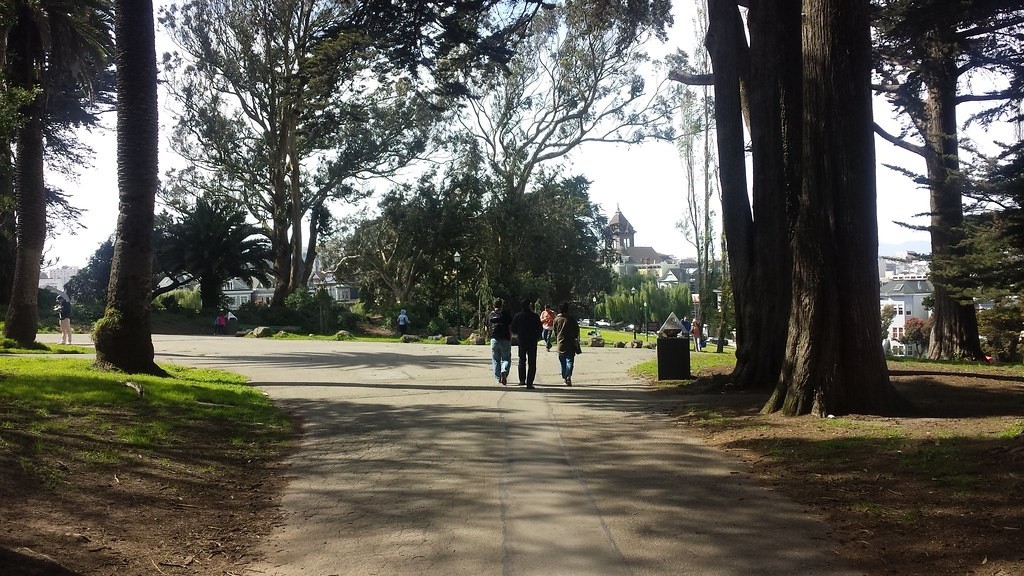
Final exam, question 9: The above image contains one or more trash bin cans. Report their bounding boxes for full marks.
[656,311,692,379]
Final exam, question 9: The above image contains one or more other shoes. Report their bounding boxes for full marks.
[214,333,215,336]
[501,372,507,385]
[518,382,525,385]
[527,385,534,389]
[549,345,552,349]
[546,348,549,352]
[57,343,65,345]
[497,380,501,383]
[566,379,571,386]
[66,342,71,345]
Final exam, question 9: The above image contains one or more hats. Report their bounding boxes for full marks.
[55,295,63,301]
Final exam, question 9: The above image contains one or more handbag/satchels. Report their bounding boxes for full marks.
[572,340,582,354]
[699,341,706,347]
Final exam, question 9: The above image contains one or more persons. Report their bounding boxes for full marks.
[540,304,556,352]
[211,310,237,335]
[55,295,72,345]
[511,299,543,389]
[553,303,579,386]
[485,298,513,385]
[680,317,691,337]
[397,310,410,335]
[690,319,701,352]
